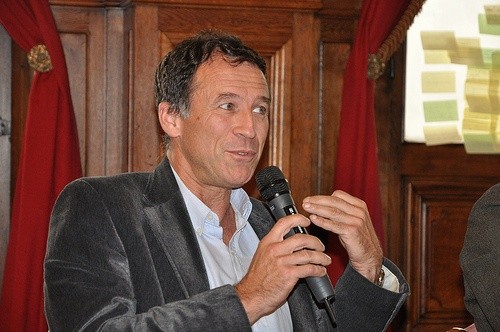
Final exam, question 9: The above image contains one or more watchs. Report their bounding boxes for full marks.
[376,269,386,289]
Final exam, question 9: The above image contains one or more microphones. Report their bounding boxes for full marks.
[255,166,338,326]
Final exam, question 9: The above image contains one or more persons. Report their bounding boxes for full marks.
[458,182,500,332]
[41,27,411,332]
